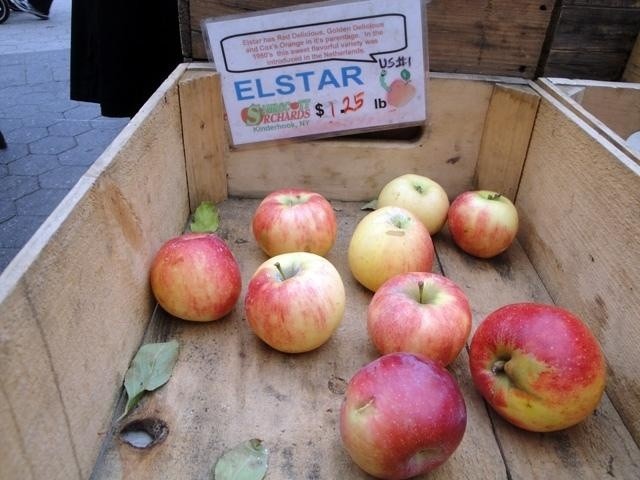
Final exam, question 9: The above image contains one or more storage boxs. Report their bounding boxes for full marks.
[531,73,638,162]
[1,61,640,480]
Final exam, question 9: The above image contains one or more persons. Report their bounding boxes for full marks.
[12,1,54,20]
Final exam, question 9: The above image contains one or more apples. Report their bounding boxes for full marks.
[149,232,242,323]
[379,173,449,236]
[386,72,418,108]
[340,352,467,480]
[251,188,337,257]
[448,191,520,258]
[469,303,608,433]
[245,252,346,354]
[368,273,472,367]
[348,206,436,294]
